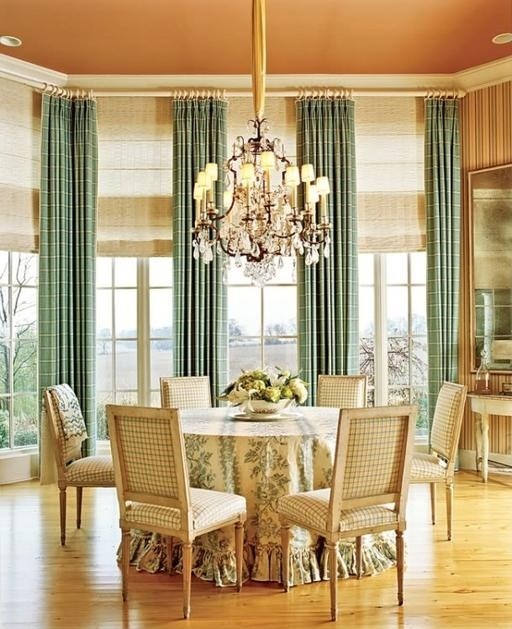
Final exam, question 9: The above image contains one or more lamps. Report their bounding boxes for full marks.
[191,0,333,288]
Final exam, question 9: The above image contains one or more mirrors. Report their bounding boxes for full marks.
[468,164,512,376]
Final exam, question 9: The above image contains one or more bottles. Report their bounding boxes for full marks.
[475,357,493,395]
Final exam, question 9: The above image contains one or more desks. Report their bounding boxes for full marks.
[467,393,512,483]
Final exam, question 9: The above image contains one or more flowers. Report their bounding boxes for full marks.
[213,366,311,407]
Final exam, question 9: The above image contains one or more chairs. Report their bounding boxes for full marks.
[278,404,417,622]
[317,375,368,408]
[45,383,116,546]
[160,375,212,410]
[105,404,247,618]
[410,381,468,541]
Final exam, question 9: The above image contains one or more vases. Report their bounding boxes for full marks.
[244,399,291,417]
